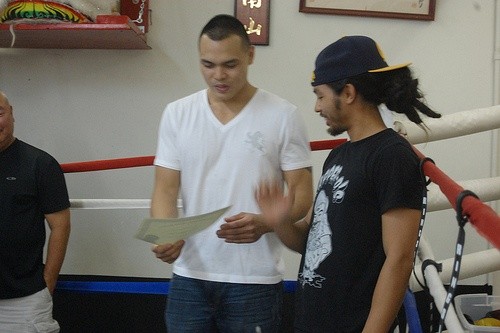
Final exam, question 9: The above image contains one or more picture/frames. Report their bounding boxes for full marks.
[299,0,436,20]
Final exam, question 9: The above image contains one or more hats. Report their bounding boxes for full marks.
[311,36,412,86]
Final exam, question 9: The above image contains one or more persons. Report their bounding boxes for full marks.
[149,13,316,333]
[249,36,441,333]
[1,92,74,333]
